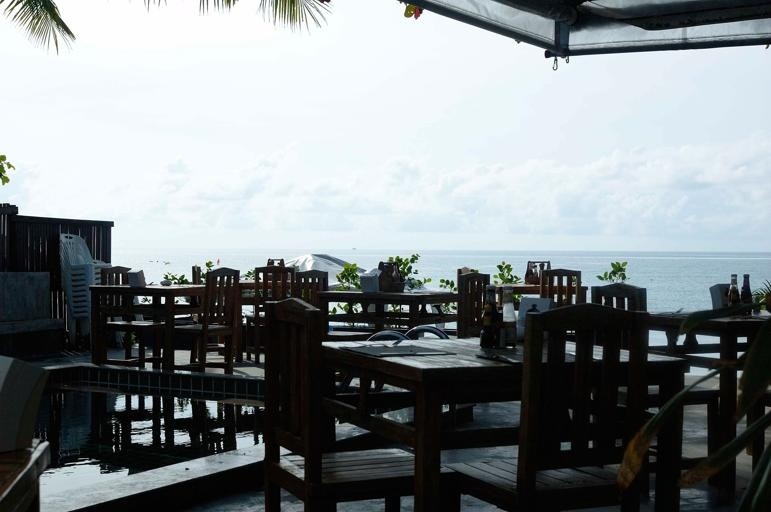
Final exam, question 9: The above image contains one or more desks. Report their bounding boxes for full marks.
[322,335,690,510]
[646,304,771,511]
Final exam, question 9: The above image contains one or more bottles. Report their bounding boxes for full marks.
[739,274,753,319]
[380,263,401,293]
[475,284,500,349]
[527,303,541,312]
[727,273,740,320]
[497,285,518,347]
[523,261,548,286]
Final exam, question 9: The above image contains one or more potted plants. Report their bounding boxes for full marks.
[377,253,431,291]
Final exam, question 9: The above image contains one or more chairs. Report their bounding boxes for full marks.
[262,297,464,511]
[590,283,651,355]
[432,302,651,511]
[0,267,771,378]
[0,358,52,512]
